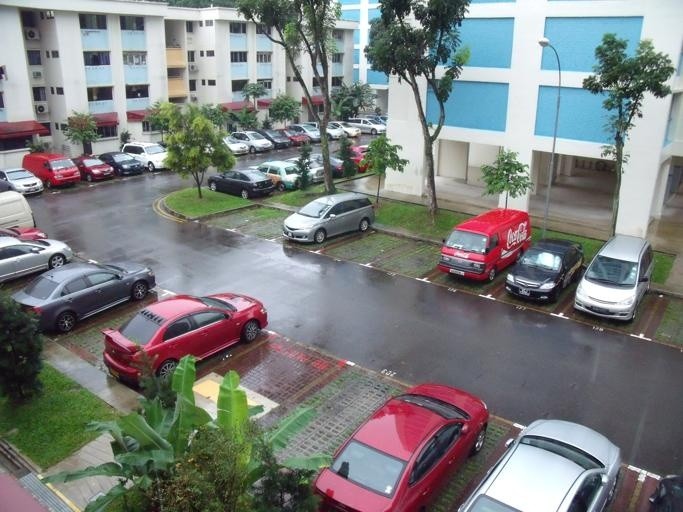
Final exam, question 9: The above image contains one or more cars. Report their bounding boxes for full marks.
[647,476,682,512]
[256,129,291,150]
[203,136,250,155]
[0,235,72,289]
[71,157,115,183]
[100,293,269,386]
[248,160,313,192]
[0,226,48,241]
[207,169,275,200]
[9,261,158,333]
[349,145,373,173]
[0,168,44,194]
[503,237,585,306]
[285,115,388,145]
[275,128,311,147]
[98,151,145,176]
[452,418,622,512]
[284,156,324,183]
[307,380,486,512]
[309,152,358,176]
[230,130,273,154]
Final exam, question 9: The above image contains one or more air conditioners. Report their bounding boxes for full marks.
[189,64,197,73]
[35,103,48,114]
[25,27,39,41]
[33,71,41,78]
[191,93,197,97]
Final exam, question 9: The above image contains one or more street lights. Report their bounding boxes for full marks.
[537,31,563,240]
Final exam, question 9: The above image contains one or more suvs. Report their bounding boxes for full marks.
[121,141,179,172]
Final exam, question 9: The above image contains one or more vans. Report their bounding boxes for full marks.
[437,206,532,284]
[1,190,35,230]
[283,191,377,243]
[21,152,81,190]
[573,233,655,322]
